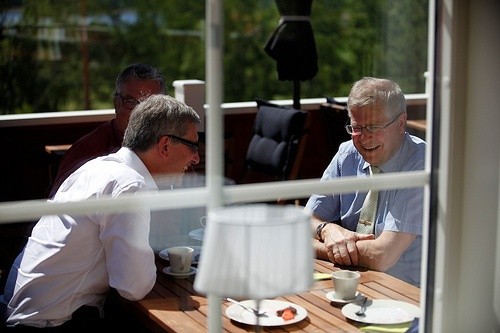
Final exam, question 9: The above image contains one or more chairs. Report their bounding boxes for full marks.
[240,100,312,204]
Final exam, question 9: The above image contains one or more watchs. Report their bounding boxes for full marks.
[316,222,329,243]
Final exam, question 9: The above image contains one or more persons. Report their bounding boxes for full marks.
[49,64,165,198]
[0,94,201,333]
[305,77,425,288]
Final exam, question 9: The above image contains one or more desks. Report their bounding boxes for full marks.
[111,256,420,333]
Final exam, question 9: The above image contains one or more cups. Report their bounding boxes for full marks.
[167,246,195,272]
[332,270,360,299]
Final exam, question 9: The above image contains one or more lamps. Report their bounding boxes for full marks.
[194,204,316,333]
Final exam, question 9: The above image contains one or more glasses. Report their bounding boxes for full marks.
[344,112,407,135]
[163,132,201,152]
[118,93,145,110]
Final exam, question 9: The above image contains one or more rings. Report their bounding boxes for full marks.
[333,251,339,255]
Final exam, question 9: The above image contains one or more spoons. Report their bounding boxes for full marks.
[227,298,267,315]
[355,296,368,316]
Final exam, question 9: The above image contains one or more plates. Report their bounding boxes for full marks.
[225,299,307,325]
[341,299,420,325]
[159,246,204,264]
[326,291,362,304]
[161,265,198,278]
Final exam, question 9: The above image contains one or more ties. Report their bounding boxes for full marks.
[355,166,381,235]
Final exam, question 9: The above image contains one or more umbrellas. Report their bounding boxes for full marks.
[264,0,319,110]
